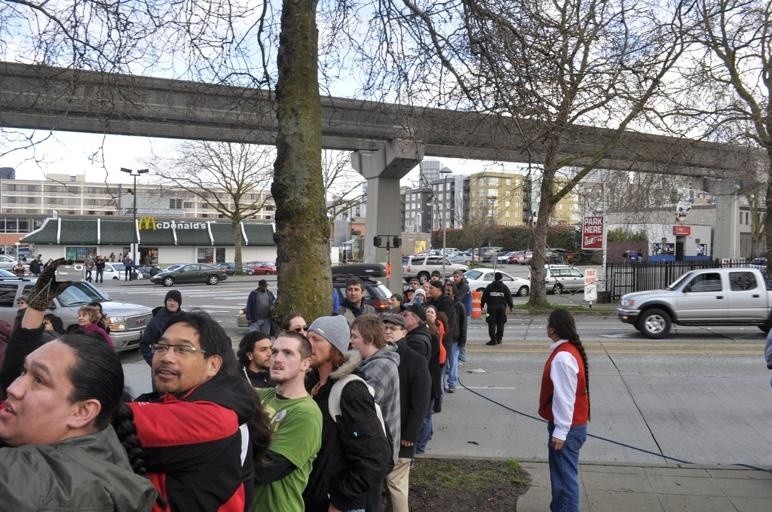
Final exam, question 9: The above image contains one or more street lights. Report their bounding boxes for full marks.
[120,167,150,274]
[438,166,454,286]
[487,196,497,224]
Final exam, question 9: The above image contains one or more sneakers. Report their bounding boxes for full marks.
[486,339,502,345]
[447,387,455,393]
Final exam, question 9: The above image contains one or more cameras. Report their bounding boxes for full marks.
[54,264,86,282]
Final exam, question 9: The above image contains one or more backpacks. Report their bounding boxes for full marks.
[329,373,394,475]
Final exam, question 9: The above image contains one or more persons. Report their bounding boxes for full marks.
[623,248,643,265]
[481,273,514,345]
[697,244,704,255]
[0,250,471,511]
[538,309,590,512]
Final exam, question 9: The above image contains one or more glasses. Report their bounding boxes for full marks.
[149,343,208,355]
[295,327,308,331]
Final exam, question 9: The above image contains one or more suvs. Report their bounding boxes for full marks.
[401,253,468,285]
[0,275,158,358]
[328,262,393,309]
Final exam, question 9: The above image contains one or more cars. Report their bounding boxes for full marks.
[150,263,228,285]
[748,258,767,273]
[0,253,279,280]
[448,267,530,297]
[0,268,18,278]
[417,246,571,264]
[527,263,586,294]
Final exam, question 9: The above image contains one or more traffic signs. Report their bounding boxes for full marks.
[582,215,603,251]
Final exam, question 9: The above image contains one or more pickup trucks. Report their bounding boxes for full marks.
[615,267,772,339]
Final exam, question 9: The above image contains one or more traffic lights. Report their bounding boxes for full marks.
[374,236,381,246]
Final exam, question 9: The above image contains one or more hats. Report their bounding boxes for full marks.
[403,303,426,321]
[417,271,443,289]
[259,279,269,286]
[309,314,351,355]
[382,313,407,327]
[164,290,181,308]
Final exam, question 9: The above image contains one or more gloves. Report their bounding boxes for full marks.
[26,257,73,310]
